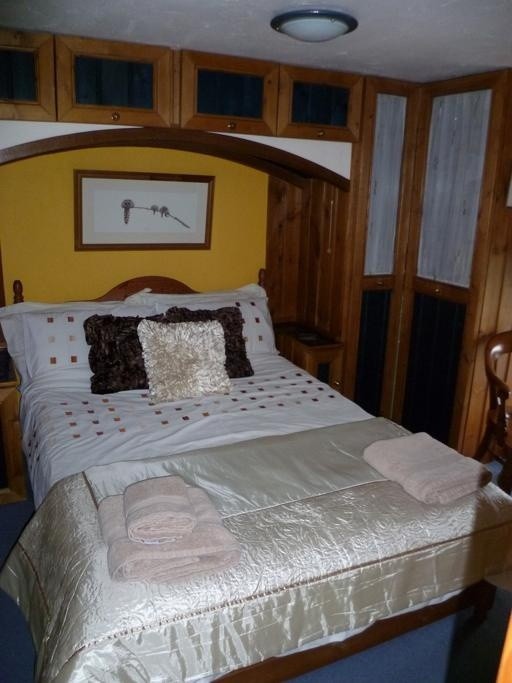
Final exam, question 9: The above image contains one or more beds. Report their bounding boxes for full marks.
[1,277,512,682]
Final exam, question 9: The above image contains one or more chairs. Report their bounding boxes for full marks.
[471,329,512,492]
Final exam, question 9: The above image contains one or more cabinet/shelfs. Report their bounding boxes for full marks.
[0,29,363,152]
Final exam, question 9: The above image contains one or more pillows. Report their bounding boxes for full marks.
[0,283,281,404]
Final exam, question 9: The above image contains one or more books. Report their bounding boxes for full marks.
[295,332,337,347]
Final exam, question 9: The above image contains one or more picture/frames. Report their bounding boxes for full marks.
[74,167,215,252]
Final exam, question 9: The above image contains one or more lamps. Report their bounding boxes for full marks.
[270,8,359,43]
